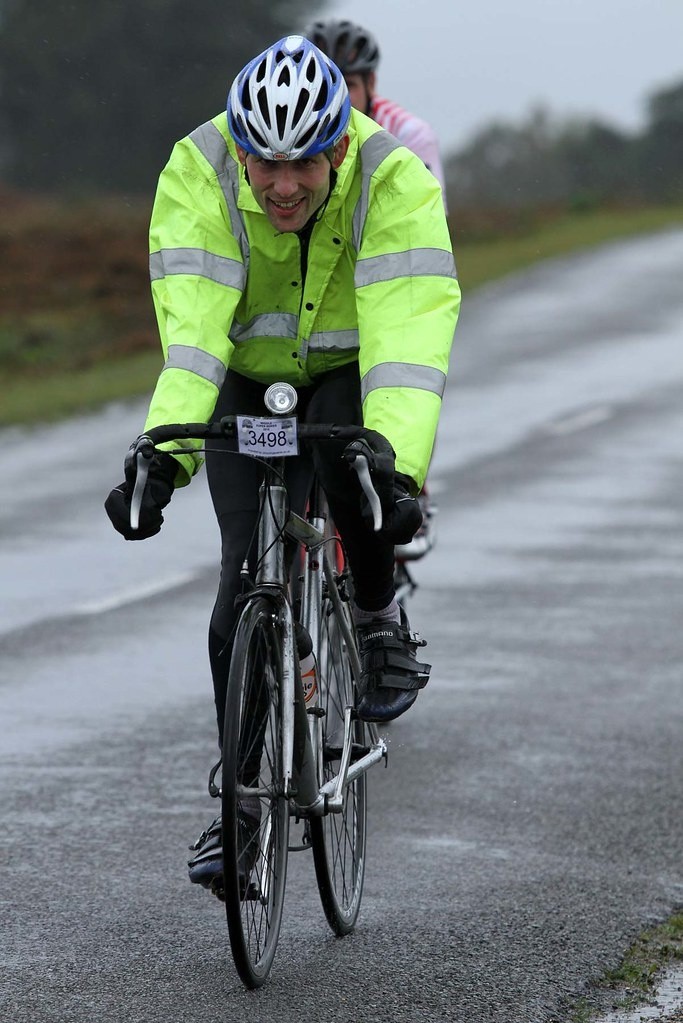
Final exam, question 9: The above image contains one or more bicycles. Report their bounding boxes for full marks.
[124,382,439,989]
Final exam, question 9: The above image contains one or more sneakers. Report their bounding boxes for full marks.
[395,498,438,559]
[353,602,434,721]
[187,808,260,882]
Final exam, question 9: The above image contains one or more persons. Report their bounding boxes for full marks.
[304,20,447,560]
[104,36,461,882]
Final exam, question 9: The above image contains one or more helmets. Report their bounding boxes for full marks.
[303,18,380,75]
[225,33,352,162]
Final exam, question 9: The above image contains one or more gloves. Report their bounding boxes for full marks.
[103,463,174,541]
[363,481,422,544]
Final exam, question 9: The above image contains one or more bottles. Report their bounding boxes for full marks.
[265,622,319,712]
